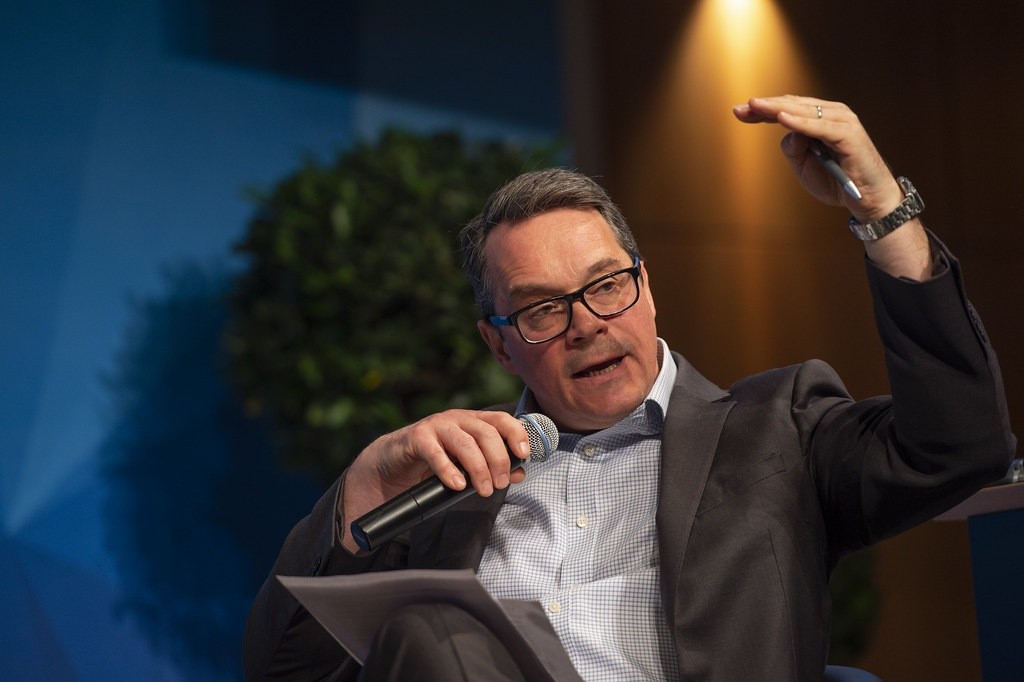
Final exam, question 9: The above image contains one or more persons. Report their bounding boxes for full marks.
[244,92,1016,682]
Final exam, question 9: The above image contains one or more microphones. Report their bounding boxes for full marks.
[350,412,560,552]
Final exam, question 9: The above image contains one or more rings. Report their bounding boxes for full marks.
[817,106,822,118]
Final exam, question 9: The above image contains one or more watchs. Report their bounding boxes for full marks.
[848,176,927,242]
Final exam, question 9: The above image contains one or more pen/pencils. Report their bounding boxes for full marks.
[808,140,862,201]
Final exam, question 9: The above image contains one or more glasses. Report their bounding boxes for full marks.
[490,255,640,344]
